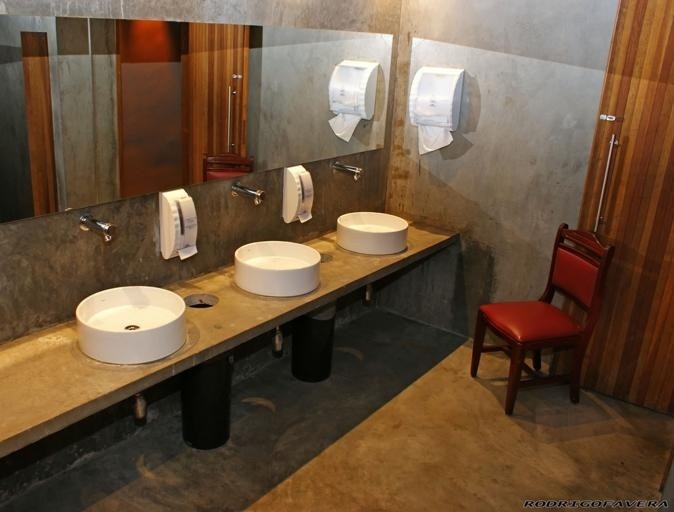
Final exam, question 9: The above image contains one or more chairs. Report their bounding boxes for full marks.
[469,222,615,415]
[200,151,254,182]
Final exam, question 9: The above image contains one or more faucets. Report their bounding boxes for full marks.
[231,181,266,208]
[331,160,364,182]
[78,214,114,243]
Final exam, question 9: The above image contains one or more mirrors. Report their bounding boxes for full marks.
[0,13,393,230]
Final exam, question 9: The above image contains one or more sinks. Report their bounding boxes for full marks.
[235,240,321,297]
[336,211,409,255]
[76,285,188,364]
